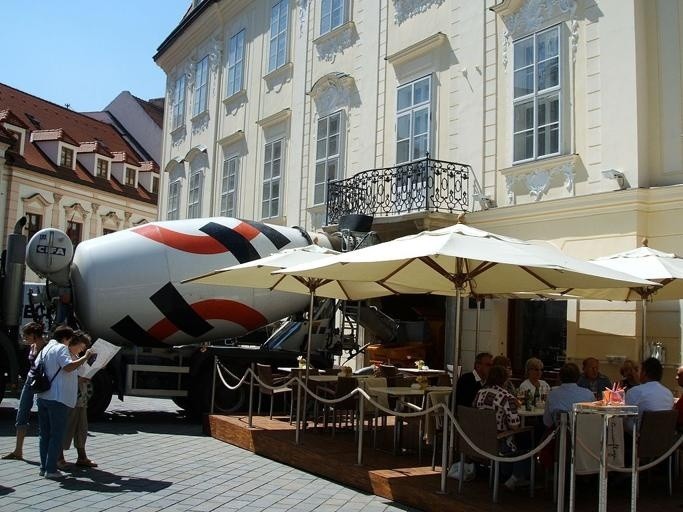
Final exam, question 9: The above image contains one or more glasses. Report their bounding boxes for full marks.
[530,367,544,372]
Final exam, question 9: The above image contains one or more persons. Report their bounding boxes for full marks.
[57,328,97,468]
[449,352,683,492]
[28,323,94,483]
[1,322,50,460]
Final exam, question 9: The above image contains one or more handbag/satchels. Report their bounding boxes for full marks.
[447,461,476,482]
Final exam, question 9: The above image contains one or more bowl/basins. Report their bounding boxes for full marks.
[535,402,545,409]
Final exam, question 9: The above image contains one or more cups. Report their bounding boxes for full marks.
[601,390,626,406]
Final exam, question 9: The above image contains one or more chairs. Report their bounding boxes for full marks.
[257,363,368,426]
[424,379,682,501]
[358,364,462,458]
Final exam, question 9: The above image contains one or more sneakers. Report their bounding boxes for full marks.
[1,452,25,460]
[75,460,98,469]
[504,474,532,492]
[39,461,72,482]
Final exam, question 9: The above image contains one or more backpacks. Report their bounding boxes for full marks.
[24,342,62,394]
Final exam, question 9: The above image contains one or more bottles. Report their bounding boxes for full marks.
[526,388,532,411]
[337,365,353,378]
[534,384,549,407]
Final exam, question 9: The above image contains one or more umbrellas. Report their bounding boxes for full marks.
[270,212,664,468]
[179,237,430,435]
[532,236,683,385]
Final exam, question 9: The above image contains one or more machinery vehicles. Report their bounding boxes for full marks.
[364,295,446,369]
[0,214,401,439]
